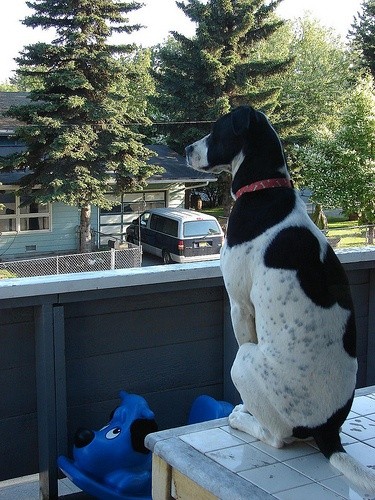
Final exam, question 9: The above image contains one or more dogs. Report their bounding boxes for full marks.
[185,105,375,500]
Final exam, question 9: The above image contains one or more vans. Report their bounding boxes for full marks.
[125,208,226,264]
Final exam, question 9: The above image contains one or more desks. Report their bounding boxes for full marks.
[144,385,375,500]
